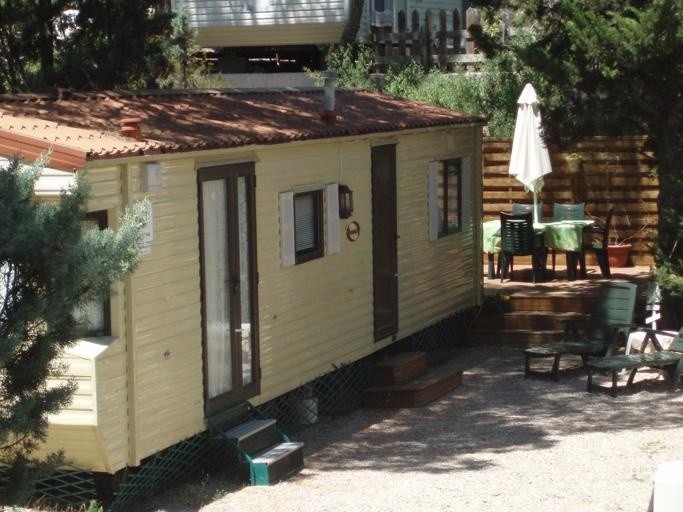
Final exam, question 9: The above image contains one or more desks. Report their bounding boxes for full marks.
[483,219,597,281]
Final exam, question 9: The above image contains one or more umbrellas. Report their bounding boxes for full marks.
[509,84,552,223]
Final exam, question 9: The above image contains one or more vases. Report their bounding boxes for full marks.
[607,243,632,268]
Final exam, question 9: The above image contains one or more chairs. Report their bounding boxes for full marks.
[582,208,614,278]
[511,202,543,272]
[583,326,683,398]
[521,280,638,382]
[500,211,546,284]
[552,202,587,273]
